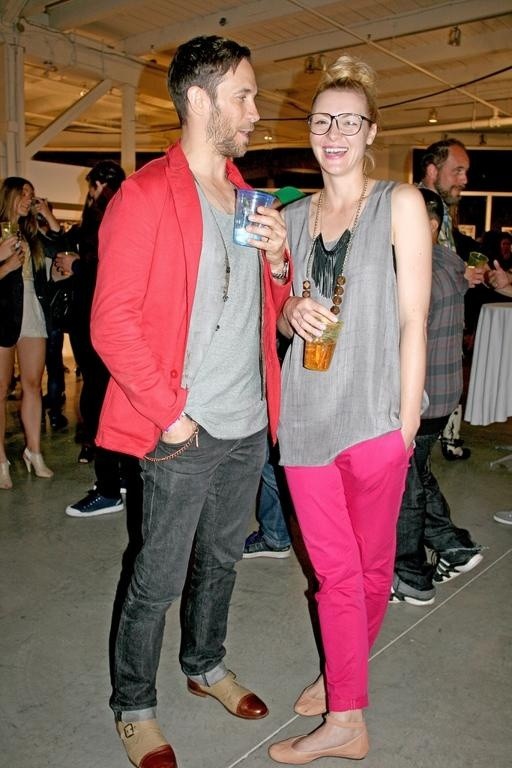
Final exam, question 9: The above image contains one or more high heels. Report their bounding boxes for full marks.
[23,449,54,478]
[0,460,12,489]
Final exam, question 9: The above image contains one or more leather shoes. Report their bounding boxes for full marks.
[188,663,368,764]
[117,719,177,768]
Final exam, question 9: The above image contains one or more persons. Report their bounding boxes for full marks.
[269,76,433,766]
[88,34,295,764]
[1,159,144,517]
[239,139,509,606]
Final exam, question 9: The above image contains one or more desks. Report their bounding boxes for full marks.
[480,303,512,526]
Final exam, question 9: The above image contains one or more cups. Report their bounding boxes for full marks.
[1,221,22,256]
[58,252,75,276]
[232,189,277,247]
[303,322,341,370]
[32,194,48,220]
[468,251,489,271]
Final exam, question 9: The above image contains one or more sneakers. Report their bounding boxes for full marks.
[388,510,512,605]
[66,447,131,517]
[242,531,291,558]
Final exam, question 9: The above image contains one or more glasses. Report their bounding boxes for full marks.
[307,113,372,135]
